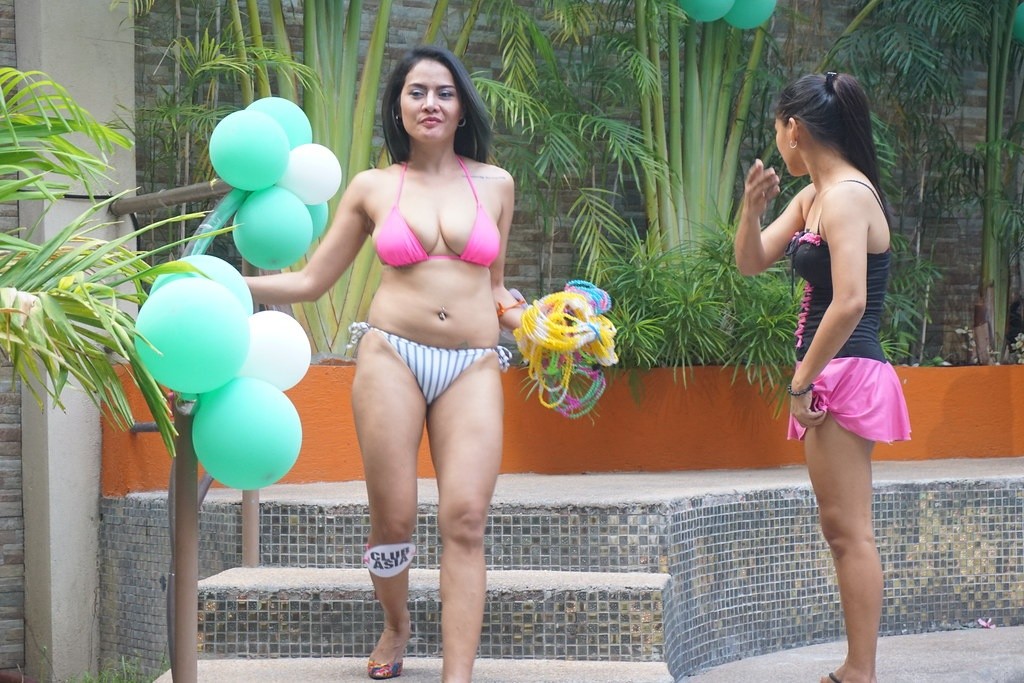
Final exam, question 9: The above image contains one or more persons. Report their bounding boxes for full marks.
[732,70,913,683]
[236,44,620,683]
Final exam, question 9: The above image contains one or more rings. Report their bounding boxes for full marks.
[761,190,765,198]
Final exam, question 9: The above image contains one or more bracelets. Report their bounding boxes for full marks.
[787,381,815,397]
